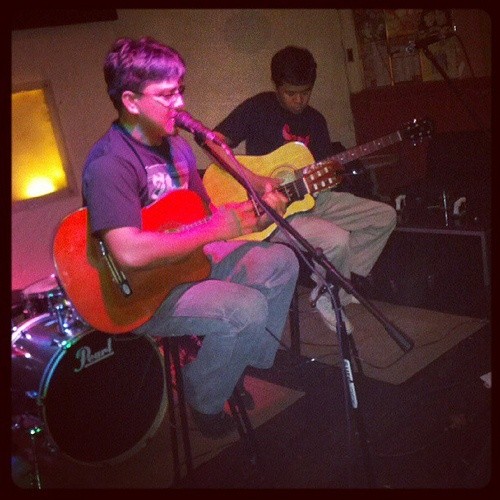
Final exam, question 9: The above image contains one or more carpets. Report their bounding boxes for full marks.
[280,285,488,385]
[8,367,308,489]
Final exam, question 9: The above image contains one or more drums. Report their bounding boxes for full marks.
[21,274,67,318]
[9,303,168,468]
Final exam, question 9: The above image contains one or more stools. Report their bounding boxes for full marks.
[142,332,261,488]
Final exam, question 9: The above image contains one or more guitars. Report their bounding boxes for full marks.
[203,112,435,241]
[51,158,356,335]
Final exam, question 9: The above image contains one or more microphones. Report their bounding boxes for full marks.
[175,110,225,147]
[405,31,451,53]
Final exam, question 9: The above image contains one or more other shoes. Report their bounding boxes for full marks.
[310,286,354,335]
[188,407,232,440]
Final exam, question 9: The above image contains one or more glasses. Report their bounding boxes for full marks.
[140,87,187,105]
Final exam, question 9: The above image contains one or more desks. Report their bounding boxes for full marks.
[354,221,493,286]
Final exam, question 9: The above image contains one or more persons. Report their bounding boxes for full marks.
[214,46,396,335]
[81,34,299,437]
